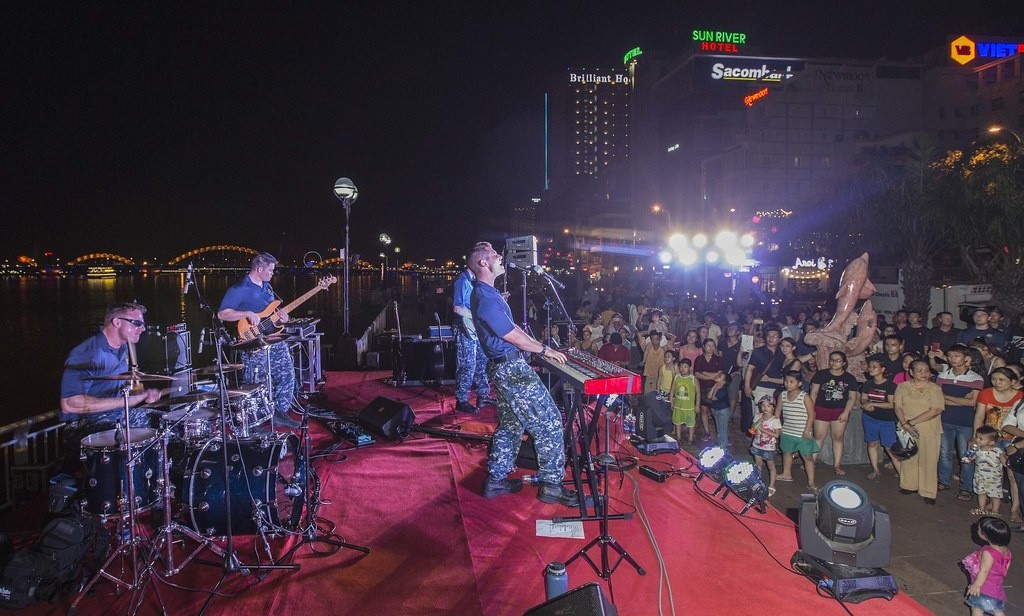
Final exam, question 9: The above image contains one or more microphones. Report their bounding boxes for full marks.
[198,328,205,353]
[510,262,531,274]
[183,264,193,294]
[533,266,566,289]
[284,486,303,497]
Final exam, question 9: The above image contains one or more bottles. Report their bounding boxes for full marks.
[623,414,636,439]
[545,562,567,599]
[963,444,978,459]
[117,517,131,556]
[522,474,539,484]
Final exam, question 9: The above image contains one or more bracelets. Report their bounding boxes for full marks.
[541,343,546,354]
[907,420,915,426]
[902,423,907,427]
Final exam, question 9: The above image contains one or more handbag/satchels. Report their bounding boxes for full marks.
[1006,436,1024,475]
[749,370,763,391]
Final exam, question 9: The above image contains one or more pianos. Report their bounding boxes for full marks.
[534,346,642,524]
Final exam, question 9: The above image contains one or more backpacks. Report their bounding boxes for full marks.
[0,510,110,609]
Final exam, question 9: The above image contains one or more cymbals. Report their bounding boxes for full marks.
[230,332,291,351]
[138,381,264,410]
[191,363,247,376]
[75,372,186,382]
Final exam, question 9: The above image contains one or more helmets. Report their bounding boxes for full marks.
[890,439,919,460]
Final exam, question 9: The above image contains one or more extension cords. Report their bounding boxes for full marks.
[638,464,665,483]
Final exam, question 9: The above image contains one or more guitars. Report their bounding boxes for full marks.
[461,291,511,341]
[237,273,338,353]
[392,300,408,382]
[431,310,445,380]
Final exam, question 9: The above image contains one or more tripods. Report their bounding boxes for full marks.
[565,412,647,581]
[68,278,371,616]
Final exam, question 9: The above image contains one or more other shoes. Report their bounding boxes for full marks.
[925,497,936,505]
[761,452,845,497]
[952,472,960,480]
[669,422,753,448]
[1009,505,1021,522]
[898,488,918,495]
[867,470,881,480]
[991,508,1000,518]
[151,518,191,535]
[970,507,986,515]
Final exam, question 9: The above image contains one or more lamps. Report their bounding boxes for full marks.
[635,391,673,441]
[721,459,769,515]
[693,447,737,496]
[798,480,892,567]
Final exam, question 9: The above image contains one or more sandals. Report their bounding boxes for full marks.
[957,488,972,502]
[937,482,950,490]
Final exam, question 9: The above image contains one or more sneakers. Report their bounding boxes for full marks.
[481,479,523,498]
[455,400,480,413]
[476,396,498,407]
[273,411,302,428]
[537,480,587,506]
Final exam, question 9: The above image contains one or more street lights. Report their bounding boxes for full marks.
[333,176,358,339]
[652,205,671,230]
[655,230,753,307]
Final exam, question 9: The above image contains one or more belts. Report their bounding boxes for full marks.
[489,350,526,364]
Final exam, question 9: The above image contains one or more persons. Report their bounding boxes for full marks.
[961,516,1012,616]
[527,298,537,330]
[59,302,162,513]
[218,252,302,432]
[466,242,580,505]
[542,286,1024,533]
[452,268,497,414]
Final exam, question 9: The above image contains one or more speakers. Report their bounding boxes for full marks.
[522,581,618,616]
[145,368,193,397]
[137,331,193,373]
[357,396,416,440]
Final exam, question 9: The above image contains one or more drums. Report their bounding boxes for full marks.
[159,407,222,444]
[77,427,167,519]
[179,430,307,539]
[214,383,274,429]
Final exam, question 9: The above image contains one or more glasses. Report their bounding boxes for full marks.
[829,358,845,362]
[111,317,146,327]
